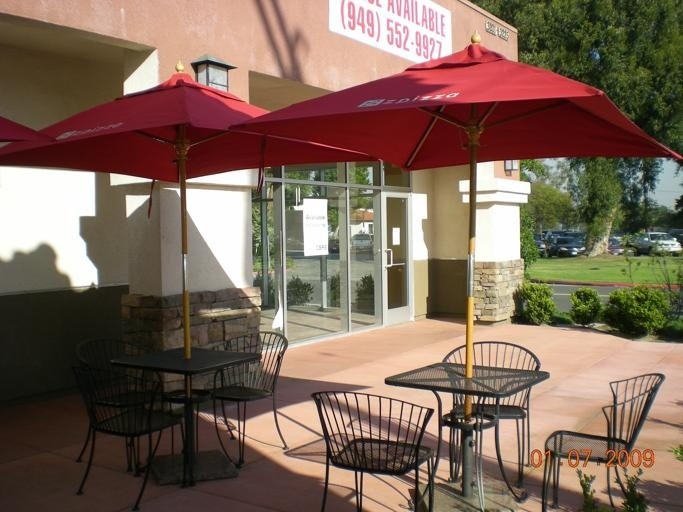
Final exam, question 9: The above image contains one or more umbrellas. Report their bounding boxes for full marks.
[0,115,55,143]
[1,59,385,465]
[234,27,682,494]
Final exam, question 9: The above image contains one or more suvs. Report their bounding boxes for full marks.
[536,233,549,259]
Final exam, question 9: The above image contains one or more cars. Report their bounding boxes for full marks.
[328,240,339,253]
[644,232,683,256]
[551,236,587,258]
[350,233,373,254]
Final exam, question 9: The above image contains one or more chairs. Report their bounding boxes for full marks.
[311,389,435,511]
[440,340,542,487]
[70,357,183,511]
[74,338,186,473]
[543,373,665,512]
[196,332,289,468]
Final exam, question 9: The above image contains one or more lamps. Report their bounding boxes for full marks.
[504,160,518,176]
[191,54,238,92]
